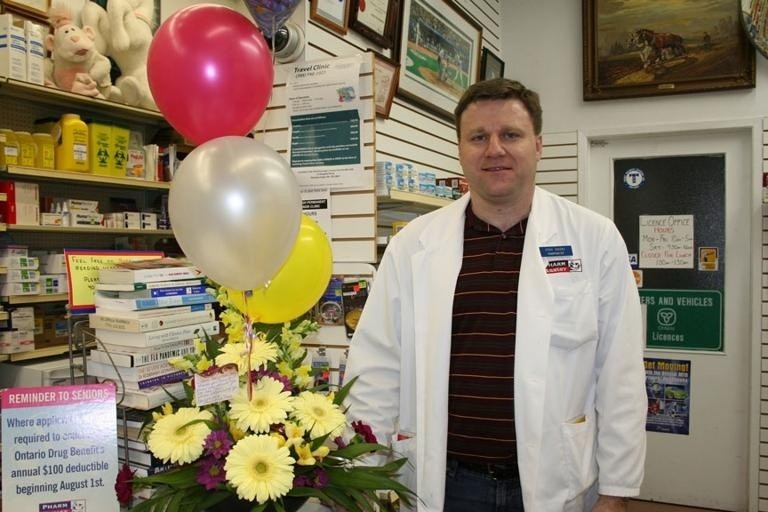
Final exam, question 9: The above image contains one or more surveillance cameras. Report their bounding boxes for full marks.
[265,23,305,64]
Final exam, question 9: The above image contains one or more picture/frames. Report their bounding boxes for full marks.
[482,46,506,81]
[350,0,401,49]
[310,0,351,36]
[366,49,401,120]
[581,0,758,102]
[738,0,768,60]
[391,0,486,126]
[0,0,57,27]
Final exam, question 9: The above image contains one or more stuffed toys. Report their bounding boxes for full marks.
[46,14,122,102]
[81,2,157,110]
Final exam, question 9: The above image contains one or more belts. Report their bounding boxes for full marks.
[445,459,521,480]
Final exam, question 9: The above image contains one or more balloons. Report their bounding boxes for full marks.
[168,135,301,294]
[224,216,332,324]
[148,5,274,144]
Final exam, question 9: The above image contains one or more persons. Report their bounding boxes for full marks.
[342,79,647,512]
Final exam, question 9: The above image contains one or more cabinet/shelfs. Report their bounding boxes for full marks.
[376,188,458,247]
[0,76,196,391]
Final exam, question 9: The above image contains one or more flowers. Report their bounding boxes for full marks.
[113,276,429,512]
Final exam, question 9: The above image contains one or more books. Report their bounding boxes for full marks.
[81,262,222,412]
[117,406,189,501]
